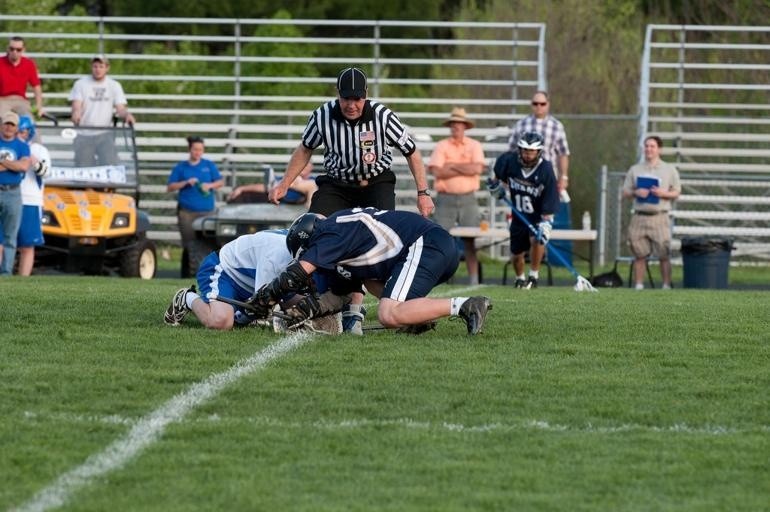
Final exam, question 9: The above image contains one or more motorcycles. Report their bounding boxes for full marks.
[189,185,313,283]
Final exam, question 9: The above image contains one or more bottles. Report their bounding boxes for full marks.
[581,211,592,231]
[192,178,210,197]
[479,212,489,230]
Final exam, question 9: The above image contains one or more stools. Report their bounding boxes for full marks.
[615,256,656,288]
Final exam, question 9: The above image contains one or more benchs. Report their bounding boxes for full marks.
[453,223,599,288]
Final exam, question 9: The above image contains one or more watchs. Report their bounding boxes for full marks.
[415,188,433,196]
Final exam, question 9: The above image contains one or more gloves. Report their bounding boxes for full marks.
[233,262,368,341]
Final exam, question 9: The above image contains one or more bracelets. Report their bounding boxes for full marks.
[560,174,570,182]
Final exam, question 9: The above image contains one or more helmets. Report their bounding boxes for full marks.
[287,212,326,253]
[515,131,546,166]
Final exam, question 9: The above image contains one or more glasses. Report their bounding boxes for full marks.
[532,101,547,106]
[8,46,23,52]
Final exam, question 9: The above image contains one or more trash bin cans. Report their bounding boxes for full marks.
[679,237,737,290]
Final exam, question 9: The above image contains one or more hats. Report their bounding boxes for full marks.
[2,110,21,126]
[91,53,112,67]
[335,67,371,98]
[440,105,476,128]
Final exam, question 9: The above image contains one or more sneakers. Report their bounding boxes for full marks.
[458,295,494,337]
[514,277,525,289]
[154,289,194,323]
[525,275,538,289]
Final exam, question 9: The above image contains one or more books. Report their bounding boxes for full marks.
[635,173,662,202]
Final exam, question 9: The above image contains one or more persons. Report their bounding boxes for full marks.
[0,114,53,276]
[505,91,571,194]
[161,225,365,337]
[0,38,45,129]
[232,205,493,337]
[228,157,318,204]
[0,111,33,275]
[620,135,683,289]
[266,66,437,299]
[68,53,136,167]
[488,129,559,289]
[426,105,487,285]
[169,136,224,277]
